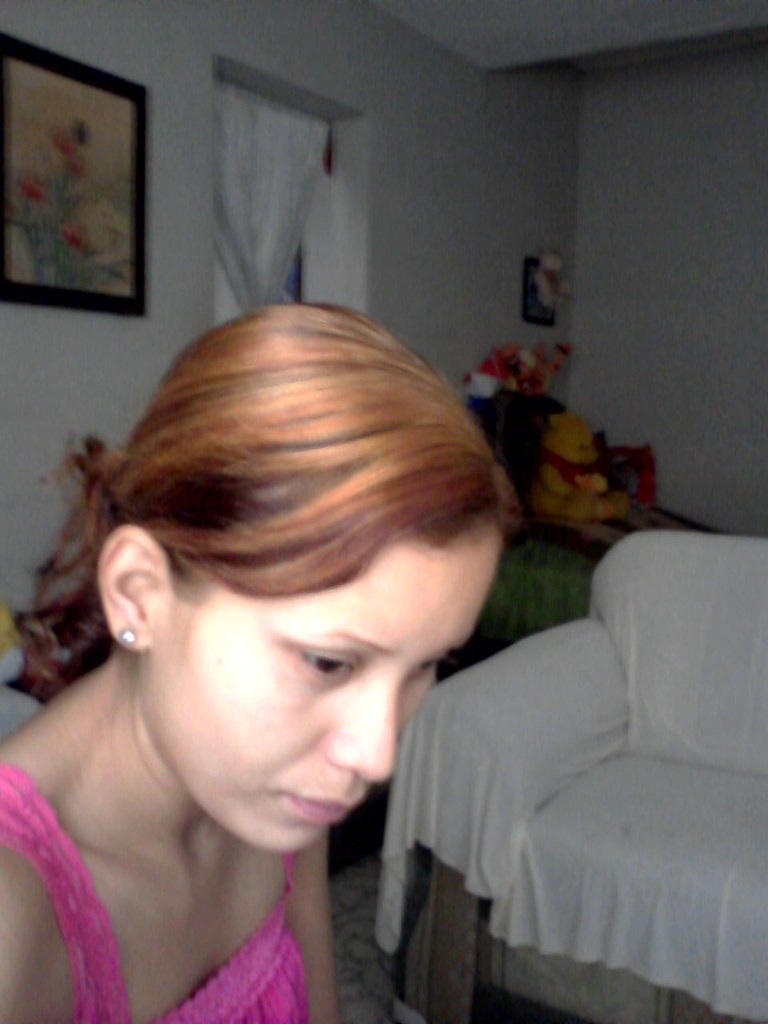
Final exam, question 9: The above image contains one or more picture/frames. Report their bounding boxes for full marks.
[0,29,149,316]
[520,257,556,324]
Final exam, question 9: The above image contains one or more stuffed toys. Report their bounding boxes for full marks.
[531,413,629,521]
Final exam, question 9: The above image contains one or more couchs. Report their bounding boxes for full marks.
[378,529,768,1024]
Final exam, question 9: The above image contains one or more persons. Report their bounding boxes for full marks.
[0,303,520,1023]
